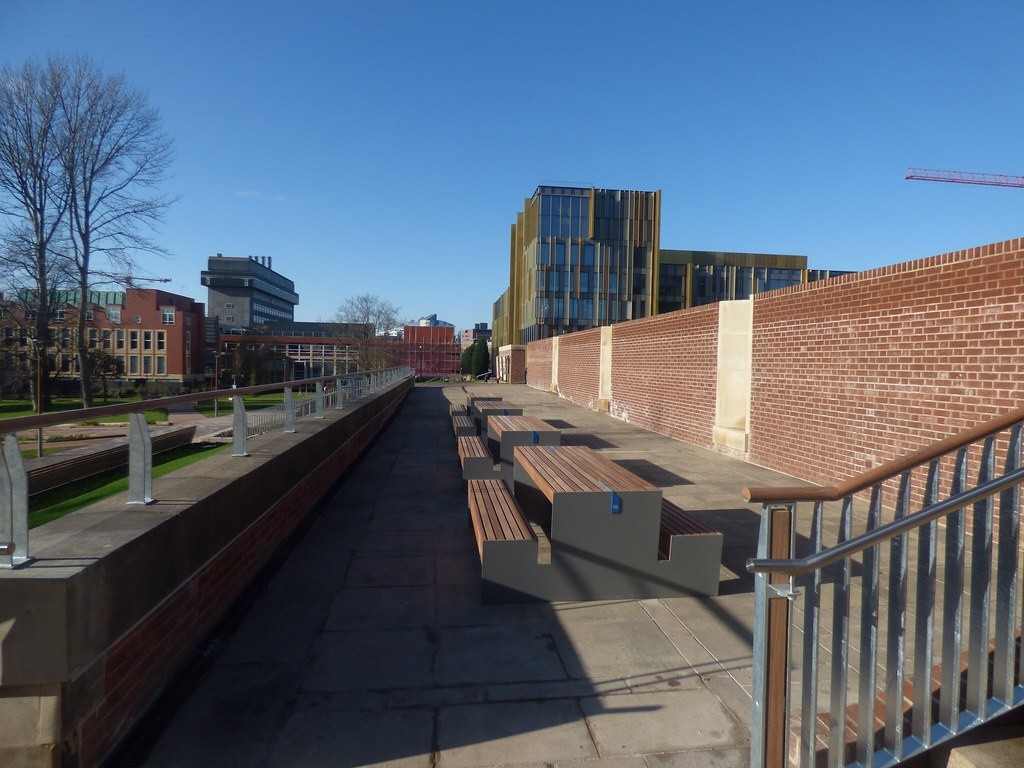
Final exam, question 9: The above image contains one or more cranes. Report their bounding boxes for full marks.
[110,261,173,288]
[904,166,1024,188]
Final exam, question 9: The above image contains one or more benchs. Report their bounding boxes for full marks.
[661,498,724,559]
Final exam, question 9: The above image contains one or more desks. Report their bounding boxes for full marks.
[449,404,540,562]
[466,391,723,604]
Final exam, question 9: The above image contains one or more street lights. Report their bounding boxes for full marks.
[212,350,221,417]
[419,344,422,380]
[281,356,289,382]
[30,338,56,458]
